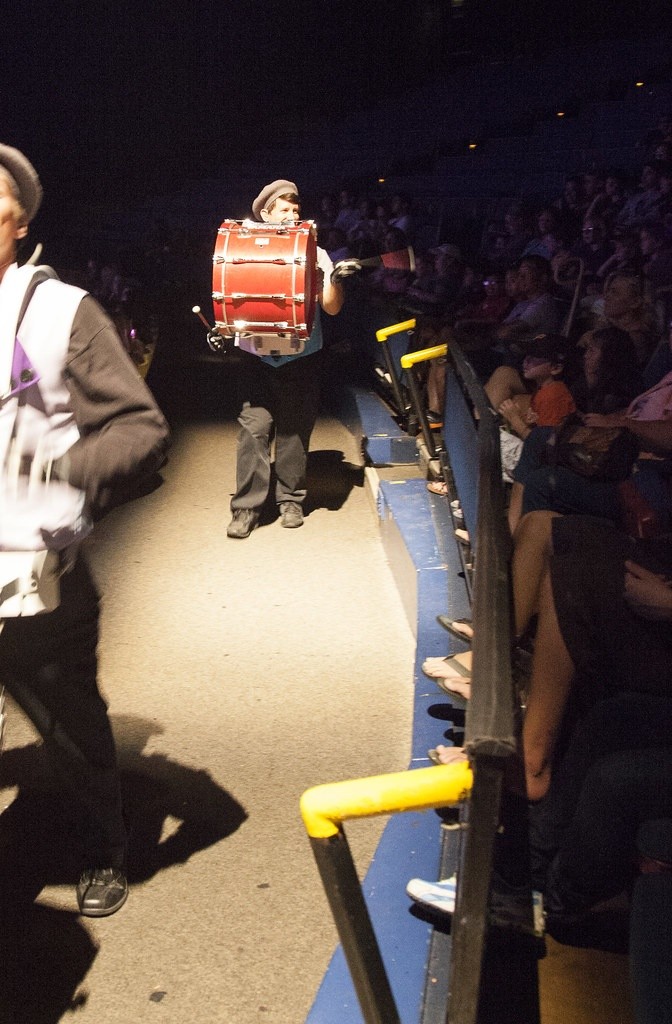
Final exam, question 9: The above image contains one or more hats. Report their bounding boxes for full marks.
[428,243,462,258]
[0,142,44,225]
[517,332,576,360]
[530,381,576,427]
[252,180,299,222]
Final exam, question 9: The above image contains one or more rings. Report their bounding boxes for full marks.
[526,417,531,420]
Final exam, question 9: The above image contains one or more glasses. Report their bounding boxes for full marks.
[482,279,501,286]
[581,226,597,232]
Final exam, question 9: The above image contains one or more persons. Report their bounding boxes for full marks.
[205,179,362,539]
[20,227,155,375]
[309,109,672,943]
[1,140,170,920]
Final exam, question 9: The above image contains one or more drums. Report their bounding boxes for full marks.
[211,217,316,358]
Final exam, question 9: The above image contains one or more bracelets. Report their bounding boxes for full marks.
[519,426,529,439]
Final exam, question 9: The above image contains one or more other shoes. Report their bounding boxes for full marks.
[384,373,392,384]
[416,411,443,429]
[453,528,470,545]
[375,368,385,378]
[427,481,449,496]
[406,872,546,938]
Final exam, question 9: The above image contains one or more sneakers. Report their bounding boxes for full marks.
[279,502,304,527]
[75,841,126,916]
[226,509,261,538]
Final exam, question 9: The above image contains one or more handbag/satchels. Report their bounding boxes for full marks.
[562,426,642,482]
[0,481,88,619]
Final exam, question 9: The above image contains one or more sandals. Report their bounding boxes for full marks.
[436,614,476,642]
[436,674,471,704]
[427,744,473,765]
[422,648,474,681]
